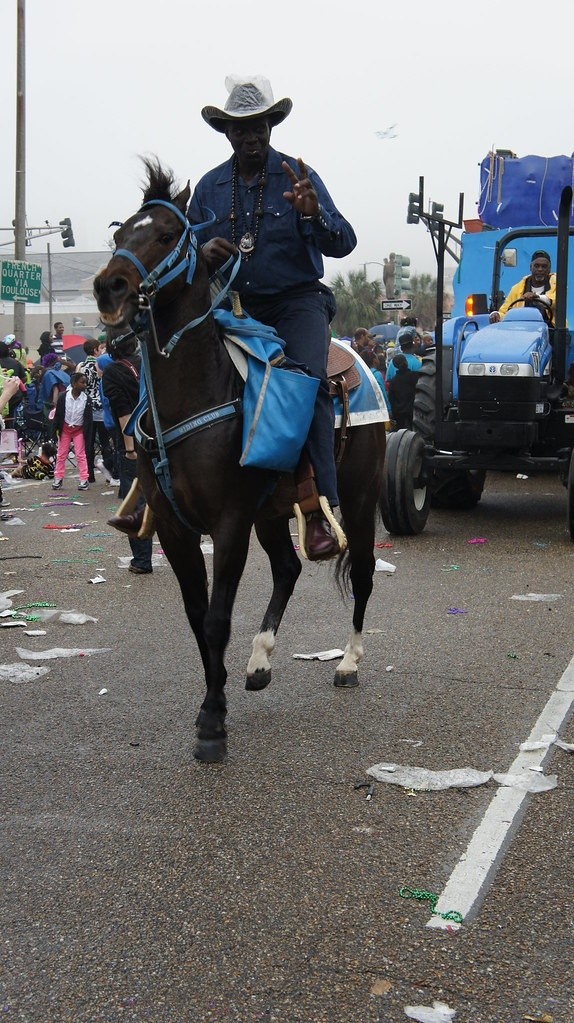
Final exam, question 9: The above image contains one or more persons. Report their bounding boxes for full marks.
[351,317,433,431]
[490,250,556,328]
[107,84,357,559]
[103,327,153,574]
[0,322,116,490]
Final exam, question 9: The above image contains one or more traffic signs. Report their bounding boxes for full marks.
[381,299,412,312]
[0,260,42,304]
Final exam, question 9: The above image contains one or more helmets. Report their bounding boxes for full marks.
[2,334,16,345]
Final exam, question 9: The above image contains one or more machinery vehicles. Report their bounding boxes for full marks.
[378,175,574,537]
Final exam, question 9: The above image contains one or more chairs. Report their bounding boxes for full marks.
[16,405,100,466]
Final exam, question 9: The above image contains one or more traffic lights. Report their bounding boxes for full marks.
[406,193,422,225]
[59,218,75,248]
[432,201,444,230]
[396,255,412,294]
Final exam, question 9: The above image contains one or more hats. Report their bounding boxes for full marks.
[367,332,376,338]
[41,354,58,368]
[340,336,353,346]
[399,334,414,346]
[201,83,293,133]
[531,250,551,263]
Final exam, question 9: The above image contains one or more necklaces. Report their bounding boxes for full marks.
[231,156,266,262]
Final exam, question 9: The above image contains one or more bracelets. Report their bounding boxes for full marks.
[126,450,134,453]
[301,203,321,220]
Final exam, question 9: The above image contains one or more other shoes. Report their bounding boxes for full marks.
[0,499,10,506]
[106,503,156,536]
[109,478,120,487]
[0,512,14,520]
[129,559,152,574]
[52,478,64,490]
[97,458,113,481]
[78,480,90,491]
[303,509,336,561]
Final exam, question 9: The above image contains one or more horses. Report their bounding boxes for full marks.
[92,159,385,764]
